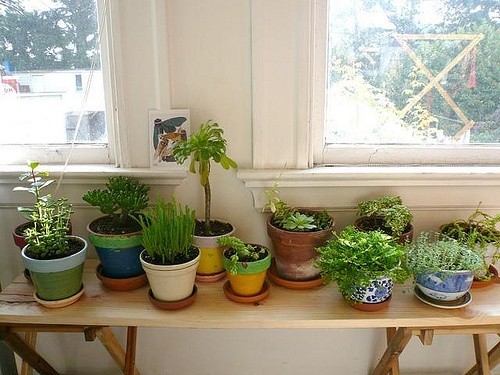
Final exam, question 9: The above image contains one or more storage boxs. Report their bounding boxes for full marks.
[66,126,105,143]
[66,109,105,128]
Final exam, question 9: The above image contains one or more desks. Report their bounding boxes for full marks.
[0,256,500,375]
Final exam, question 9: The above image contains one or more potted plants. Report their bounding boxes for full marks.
[214,235,272,304]
[10,160,73,280]
[21,194,88,308]
[406,230,494,310]
[313,223,411,310]
[172,118,238,284]
[129,194,201,311]
[262,163,336,289]
[352,195,413,248]
[82,173,152,291]
[439,201,500,289]
